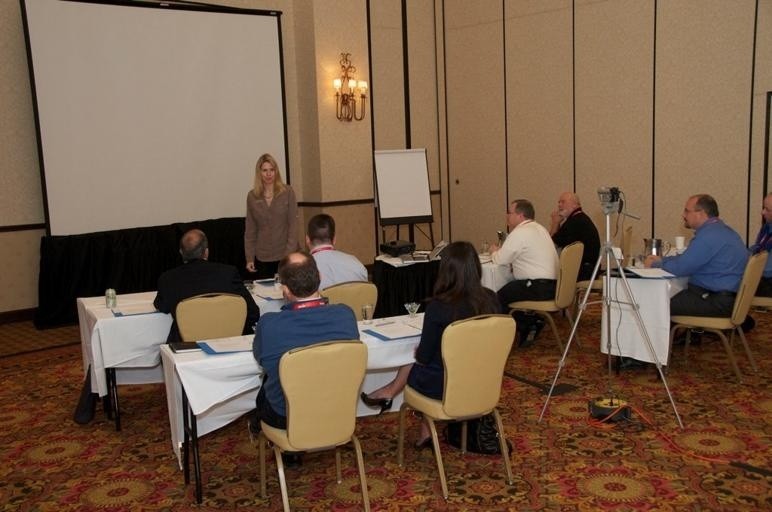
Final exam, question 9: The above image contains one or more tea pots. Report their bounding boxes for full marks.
[496,229,506,248]
[642,238,671,256]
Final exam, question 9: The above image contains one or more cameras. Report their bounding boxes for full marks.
[597,187,620,202]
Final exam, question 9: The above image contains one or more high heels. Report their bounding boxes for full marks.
[361,392,392,417]
[414,435,435,454]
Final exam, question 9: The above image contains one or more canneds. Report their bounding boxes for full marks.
[106,288,116,308]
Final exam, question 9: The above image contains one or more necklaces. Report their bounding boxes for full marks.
[264,196,273,199]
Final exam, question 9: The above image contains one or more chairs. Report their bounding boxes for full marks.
[174,291,251,345]
[508,241,591,356]
[322,280,380,322]
[662,251,772,386]
[394,313,520,502]
[256,336,373,511]
[575,275,606,319]
[574,226,635,319]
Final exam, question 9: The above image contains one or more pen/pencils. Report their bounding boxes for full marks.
[376,322,394,327]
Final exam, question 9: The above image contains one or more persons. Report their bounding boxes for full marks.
[548,192,600,282]
[734,192,772,336]
[248,252,360,467]
[607,194,749,370]
[305,213,368,290]
[244,153,300,279]
[488,199,560,348]
[360,241,502,456]
[153,229,260,343]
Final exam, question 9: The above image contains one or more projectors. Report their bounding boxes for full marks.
[380,240,416,257]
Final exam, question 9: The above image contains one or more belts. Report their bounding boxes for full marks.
[517,278,556,288]
[688,282,736,300]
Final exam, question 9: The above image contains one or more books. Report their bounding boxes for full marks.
[169,342,201,353]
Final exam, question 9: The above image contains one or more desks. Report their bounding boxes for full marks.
[374,239,513,314]
[158,308,432,503]
[596,262,690,382]
[74,278,288,434]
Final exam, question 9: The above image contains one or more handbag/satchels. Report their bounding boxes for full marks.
[75,379,99,424]
[448,415,514,455]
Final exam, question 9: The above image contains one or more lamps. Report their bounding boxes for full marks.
[331,51,372,122]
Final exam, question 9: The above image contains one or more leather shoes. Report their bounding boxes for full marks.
[519,317,545,348]
[281,453,302,468]
[610,357,648,368]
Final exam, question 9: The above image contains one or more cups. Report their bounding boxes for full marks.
[274,272,280,290]
[674,235,685,249]
[244,281,256,295]
[360,303,376,326]
[403,302,421,319]
[478,240,491,257]
[626,253,635,268]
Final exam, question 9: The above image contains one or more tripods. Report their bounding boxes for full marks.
[540,202,686,432]
